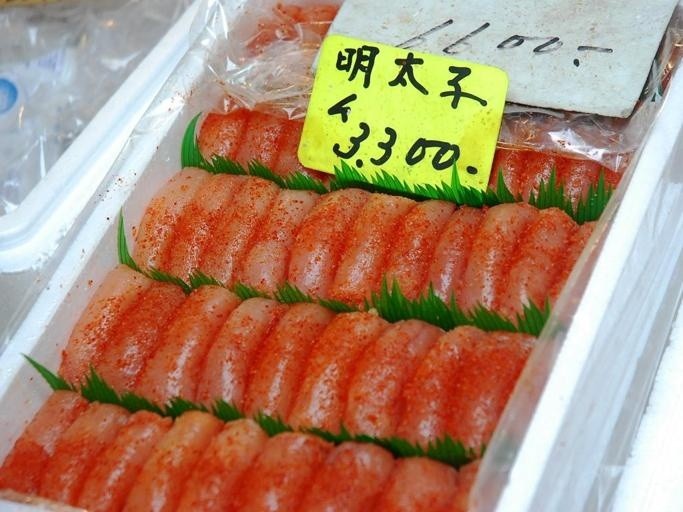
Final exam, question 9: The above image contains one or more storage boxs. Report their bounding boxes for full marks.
[0,0,683,512]
